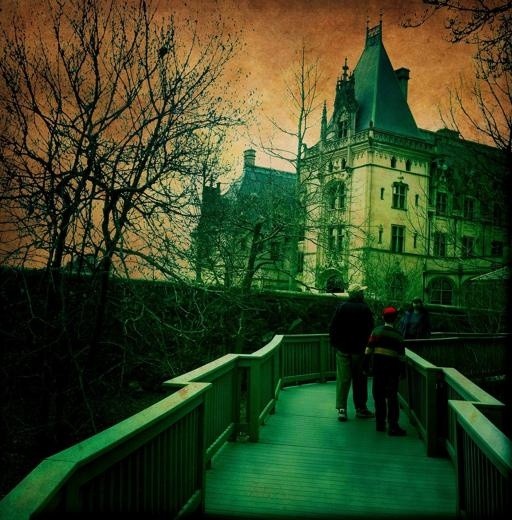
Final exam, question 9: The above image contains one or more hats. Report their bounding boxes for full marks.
[347,284,368,294]
[382,306,397,314]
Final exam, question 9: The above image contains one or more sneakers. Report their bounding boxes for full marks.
[355,407,375,418]
[335,408,348,422]
[376,424,406,436]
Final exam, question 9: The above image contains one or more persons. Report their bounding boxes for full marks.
[325,283,376,421]
[397,296,433,360]
[363,306,412,437]
